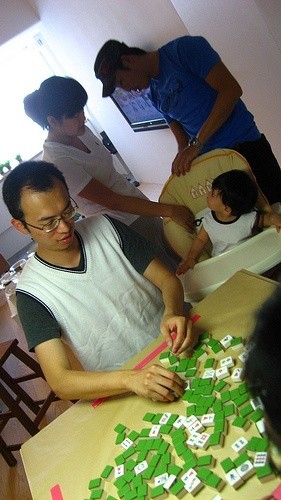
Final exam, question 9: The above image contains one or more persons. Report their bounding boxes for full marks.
[240,283,281,500]
[2,161,197,403]
[177,169,281,276]
[23,76,195,274]
[93,35,280,205]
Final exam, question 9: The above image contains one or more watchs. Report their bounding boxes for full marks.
[190,136,203,151]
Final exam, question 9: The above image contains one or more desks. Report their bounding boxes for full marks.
[20,268,281,500]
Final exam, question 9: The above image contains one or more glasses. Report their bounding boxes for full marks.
[14,195,80,233]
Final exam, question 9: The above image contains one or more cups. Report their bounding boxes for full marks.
[1,246,36,296]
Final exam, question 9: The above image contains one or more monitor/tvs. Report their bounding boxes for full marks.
[110,86,170,132]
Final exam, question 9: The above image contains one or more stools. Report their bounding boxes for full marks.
[0,338,80,467]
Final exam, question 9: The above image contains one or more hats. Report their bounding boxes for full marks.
[93,39,126,97]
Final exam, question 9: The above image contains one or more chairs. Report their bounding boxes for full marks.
[158,148,281,309]
